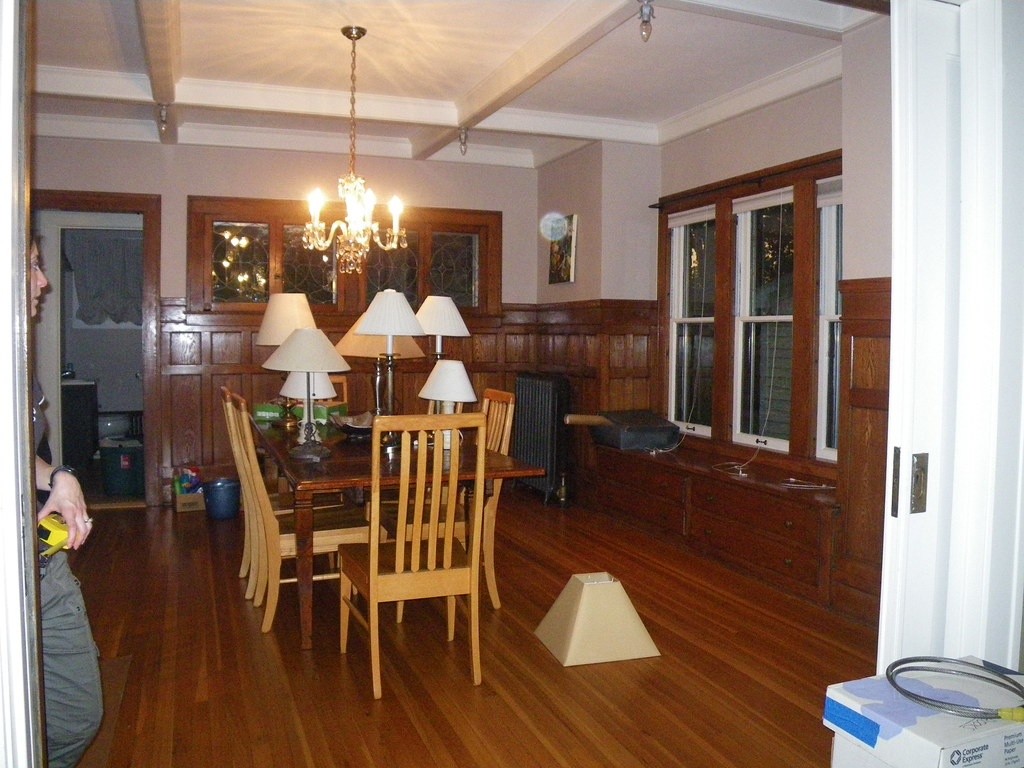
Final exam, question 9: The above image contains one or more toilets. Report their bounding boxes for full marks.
[97,413,131,442]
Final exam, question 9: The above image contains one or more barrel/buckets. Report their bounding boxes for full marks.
[203,478,240,520]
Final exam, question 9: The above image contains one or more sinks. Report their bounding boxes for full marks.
[61,379,94,385]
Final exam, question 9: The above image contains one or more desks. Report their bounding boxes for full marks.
[247,412,546,651]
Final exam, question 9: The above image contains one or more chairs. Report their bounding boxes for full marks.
[220,376,517,698]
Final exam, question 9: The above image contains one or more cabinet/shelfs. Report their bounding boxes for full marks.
[590,443,836,609]
[59,385,99,482]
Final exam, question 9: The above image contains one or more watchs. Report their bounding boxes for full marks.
[48,464,80,489]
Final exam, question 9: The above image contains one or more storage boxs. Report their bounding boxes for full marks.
[822,654,1024,768]
[595,410,680,451]
[254,400,348,425]
[172,491,206,513]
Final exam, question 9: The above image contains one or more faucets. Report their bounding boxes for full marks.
[61,371,75,379]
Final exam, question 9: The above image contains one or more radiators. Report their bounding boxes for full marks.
[513,371,570,507]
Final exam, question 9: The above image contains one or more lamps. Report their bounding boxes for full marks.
[458,126,469,155]
[636,0,655,41]
[211,228,268,301]
[256,288,478,461]
[301,26,408,274]
[158,103,170,135]
[533,572,662,666]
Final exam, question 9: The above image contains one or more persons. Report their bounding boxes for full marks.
[30,237,104,768]
